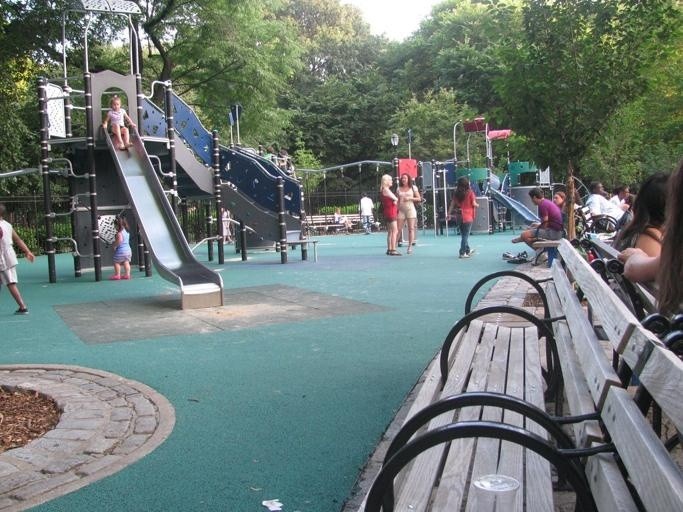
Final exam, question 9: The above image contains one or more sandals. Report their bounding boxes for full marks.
[387,250,391,254]
[390,250,402,255]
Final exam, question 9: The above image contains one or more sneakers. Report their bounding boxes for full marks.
[458,252,473,258]
[512,237,520,243]
[467,248,476,256]
[503,252,515,260]
[118,141,125,149]
[398,241,402,247]
[363,231,369,235]
[530,250,548,266]
[125,142,134,148]
[120,274,131,279]
[111,275,122,280]
[346,232,350,234]
[349,227,353,229]
[15,308,29,315]
[517,251,534,262]
[412,240,416,245]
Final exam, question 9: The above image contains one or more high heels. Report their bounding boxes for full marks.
[230,240,234,245]
[223,241,228,245]
[407,246,412,254]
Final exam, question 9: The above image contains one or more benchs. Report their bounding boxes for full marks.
[358,326,683,512]
[578,204,618,231]
[533,220,565,266]
[581,237,683,337]
[382,237,643,486]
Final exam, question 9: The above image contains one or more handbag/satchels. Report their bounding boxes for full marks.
[411,186,421,210]
[378,200,384,214]
[454,206,463,226]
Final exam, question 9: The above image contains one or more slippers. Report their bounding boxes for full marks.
[512,256,528,263]
[507,259,523,264]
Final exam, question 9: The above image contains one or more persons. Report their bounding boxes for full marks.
[512,191,567,243]
[358,192,375,235]
[102,95,136,149]
[380,174,403,255]
[617,158,683,315]
[395,173,421,254]
[521,188,563,266]
[586,182,633,228]
[446,177,479,258]
[111,214,133,279]
[609,186,630,212]
[334,208,355,234]
[222,206,235,244]
[621,170,670,257]
[0,203,35,314]
